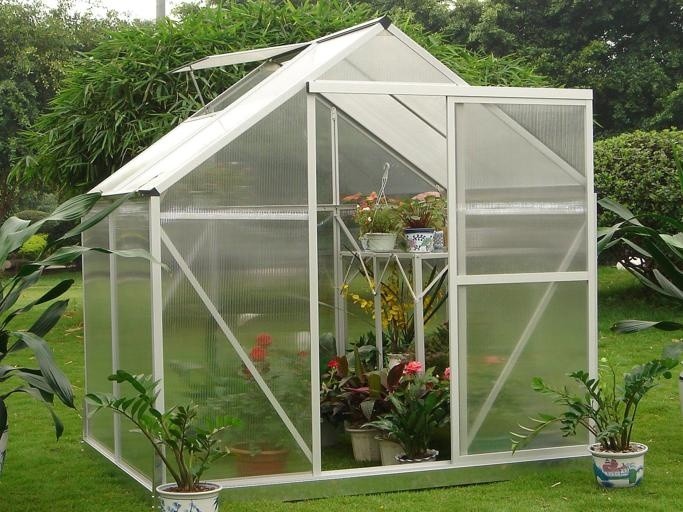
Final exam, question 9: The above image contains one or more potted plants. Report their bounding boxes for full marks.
[82,370,238,512]
[352,194,446,252]
[507,340,682,489]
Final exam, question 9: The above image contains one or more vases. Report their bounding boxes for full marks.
[229,419,438,478]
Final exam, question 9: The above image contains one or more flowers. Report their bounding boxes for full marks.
[317,263,449,410]
[341,192,408,232]
[235,325,311,388]
